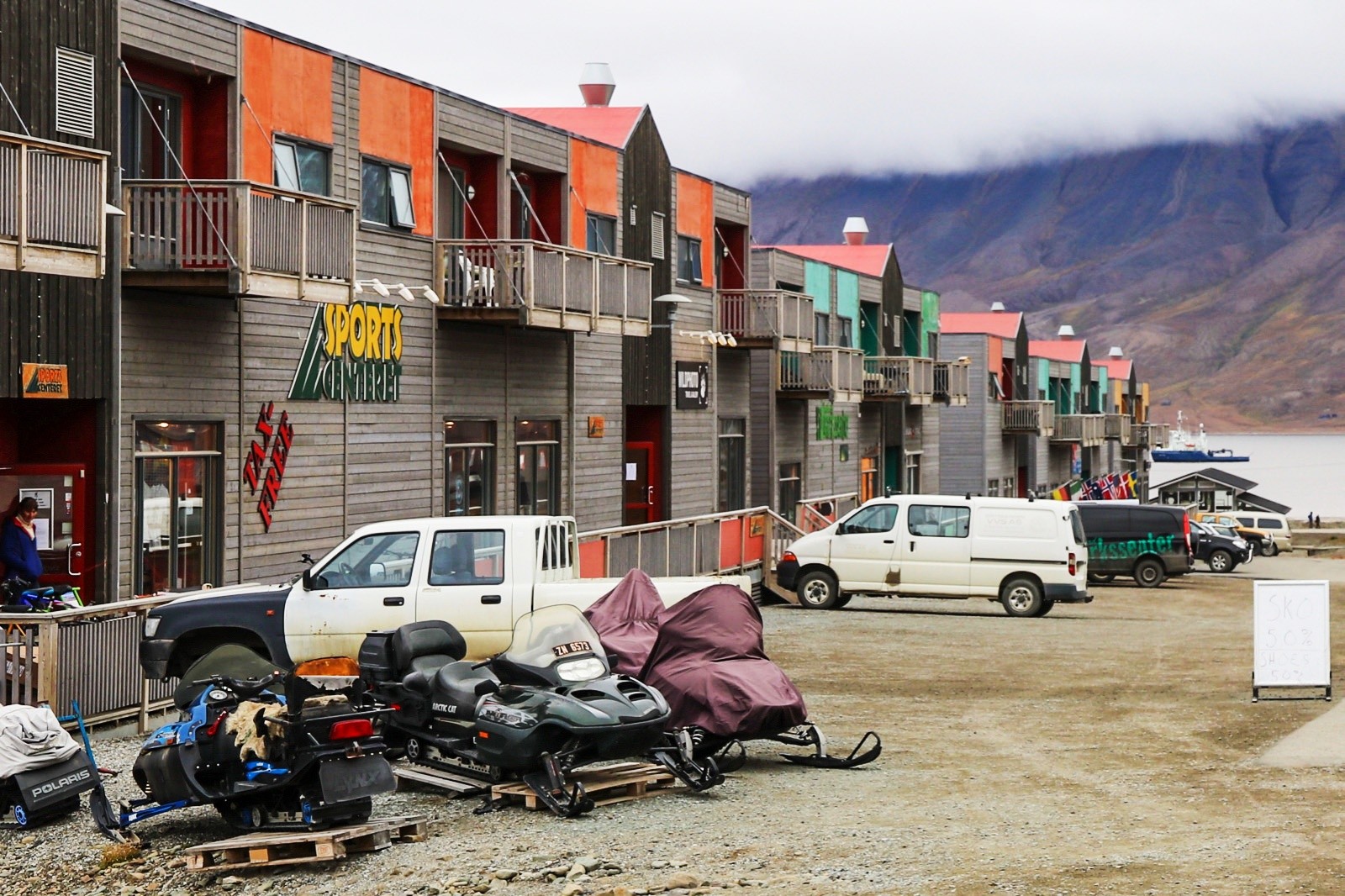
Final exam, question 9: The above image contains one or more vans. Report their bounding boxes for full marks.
[1074,505,1195,588]
[1207,512,1293,552]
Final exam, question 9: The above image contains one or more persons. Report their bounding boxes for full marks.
[0,497,44,584]
[1308,512,1313,528]
[1315,516,1320,528]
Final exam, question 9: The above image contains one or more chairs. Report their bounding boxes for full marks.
[431,547,457,584]
[458,250,500,307]
[452,543,471,584]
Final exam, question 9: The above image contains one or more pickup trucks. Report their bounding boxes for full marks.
[776,489,1094,618]
[137,514,752,682]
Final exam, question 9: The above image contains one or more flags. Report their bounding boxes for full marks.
[1053,471,1138,501]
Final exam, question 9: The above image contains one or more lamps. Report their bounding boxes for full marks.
[700,332,738,347]
[363,283,416,303]
[385,285,441,304]
[722,245,729,258]
[680,330,717,345]
[356,279,392,298]
[466,184,480,201]
[690,330,727,346]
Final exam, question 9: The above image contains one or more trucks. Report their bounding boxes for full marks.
[1149,502,1274,557]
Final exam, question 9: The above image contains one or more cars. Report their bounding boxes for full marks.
[1188,519,1253,572]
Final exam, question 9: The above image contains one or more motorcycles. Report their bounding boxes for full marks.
[116,671,399,836]
[288,603,727,817]
[581,569,882,771]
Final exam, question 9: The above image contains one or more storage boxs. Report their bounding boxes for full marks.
[356,631,400,685]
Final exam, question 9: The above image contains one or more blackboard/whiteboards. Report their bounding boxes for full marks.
[1253,583,1331,686]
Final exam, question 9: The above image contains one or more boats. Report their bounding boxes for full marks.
[1150,410,1249,462]
